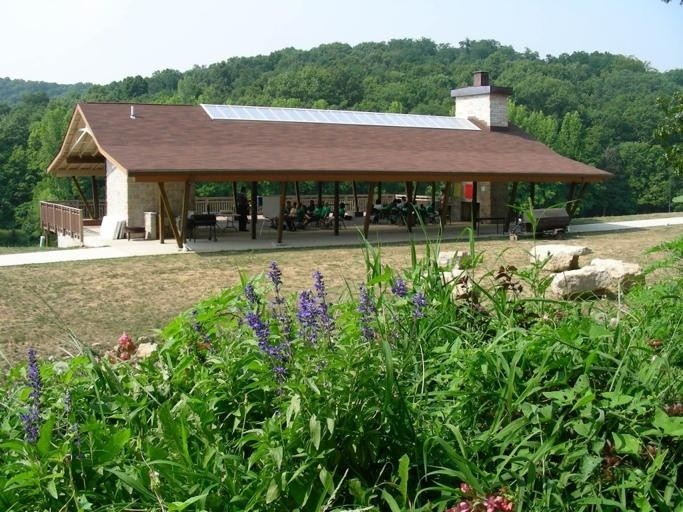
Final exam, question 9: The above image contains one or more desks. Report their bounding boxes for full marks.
[215,213,270,241]
[474,216,506,236]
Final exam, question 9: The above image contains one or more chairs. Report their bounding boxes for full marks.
[271,210,347,230]
[368,204,451,226]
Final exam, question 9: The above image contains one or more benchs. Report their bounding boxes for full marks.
[124,226,145,241]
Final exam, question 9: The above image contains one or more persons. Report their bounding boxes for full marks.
[284,199,346,231]
[373,196,432,226]
[237,186,250,232]
[440,189,447,225]
